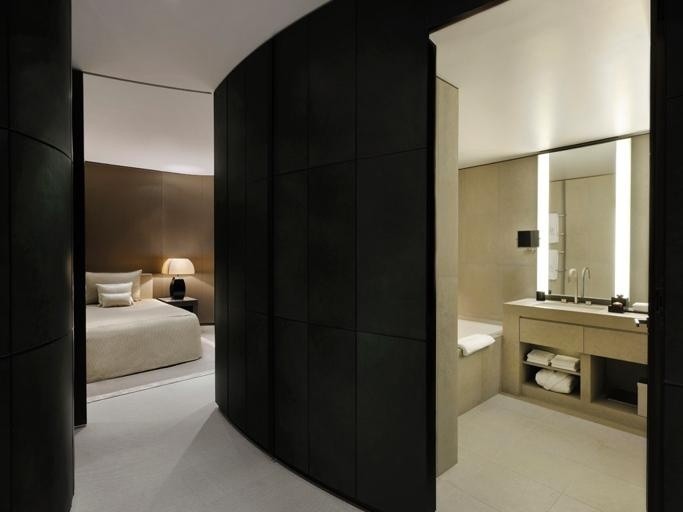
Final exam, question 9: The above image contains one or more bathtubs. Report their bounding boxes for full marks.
[456,318,503,352]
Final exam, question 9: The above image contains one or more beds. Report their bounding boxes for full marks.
[86,274,200,383]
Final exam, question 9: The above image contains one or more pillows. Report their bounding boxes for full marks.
[85,269,144,306]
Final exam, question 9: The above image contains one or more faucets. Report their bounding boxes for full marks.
[581,267,591,302]
[568,268,578,302]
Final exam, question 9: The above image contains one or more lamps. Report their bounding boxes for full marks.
[160,259,195,300]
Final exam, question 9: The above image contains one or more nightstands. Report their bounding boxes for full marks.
[160,297,199,317]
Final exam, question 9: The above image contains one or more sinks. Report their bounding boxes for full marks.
[531,301,602,314]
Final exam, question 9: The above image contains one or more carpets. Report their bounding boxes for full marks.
[86,334,217,403]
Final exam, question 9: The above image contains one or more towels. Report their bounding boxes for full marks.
[458,331,495,357]
[525,349,580,393]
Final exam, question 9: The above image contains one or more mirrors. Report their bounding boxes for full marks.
[550,140,614,302]
